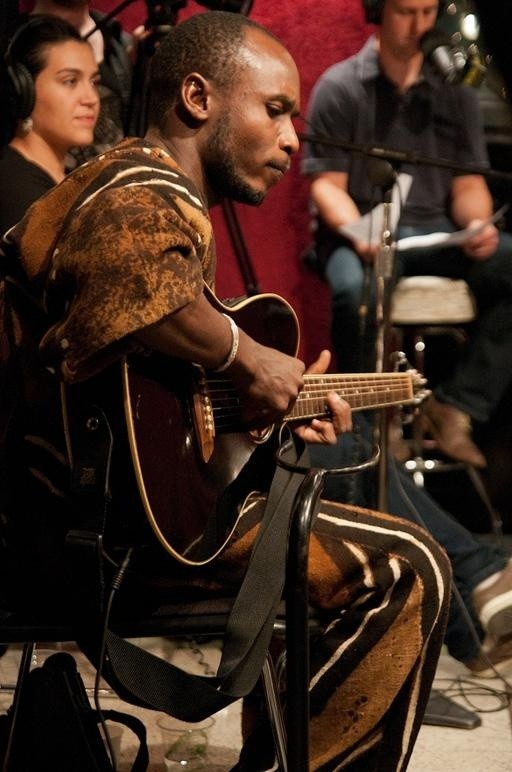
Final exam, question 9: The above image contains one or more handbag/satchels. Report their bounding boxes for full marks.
[0,652,149,771]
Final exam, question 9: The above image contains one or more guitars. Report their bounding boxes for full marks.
[62,292,428,565]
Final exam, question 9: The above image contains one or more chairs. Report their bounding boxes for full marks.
[0,270,300,770]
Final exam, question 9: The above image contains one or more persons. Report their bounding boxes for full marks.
[0,1,161,175]
[300,0,512,470]
[0,14,512,680]
[1,11,453,772]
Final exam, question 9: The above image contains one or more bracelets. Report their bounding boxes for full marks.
[213,313,239,374]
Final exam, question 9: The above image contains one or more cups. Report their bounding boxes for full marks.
[155,714,215,771]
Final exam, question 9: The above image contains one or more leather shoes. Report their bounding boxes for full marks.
[416,393,488,469]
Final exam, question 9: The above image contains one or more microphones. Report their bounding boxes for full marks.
[418,28,461,95]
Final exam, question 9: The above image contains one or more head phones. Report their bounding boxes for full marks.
[0,13,79,122]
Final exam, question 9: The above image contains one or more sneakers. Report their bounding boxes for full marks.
[462,557,511,672]
